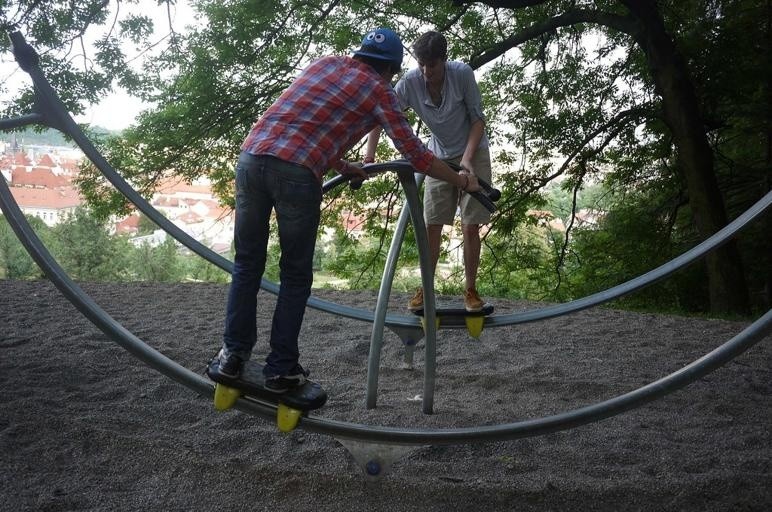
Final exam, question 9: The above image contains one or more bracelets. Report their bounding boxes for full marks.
[460,175,469,191]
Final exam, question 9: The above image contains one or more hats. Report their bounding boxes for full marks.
[353,28,405,65]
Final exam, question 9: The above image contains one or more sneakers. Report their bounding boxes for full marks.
[205,347,249,381]
[460,287,495,316]
[263,368,326,410]
[407,287,425,313]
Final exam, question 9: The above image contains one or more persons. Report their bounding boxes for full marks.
[217,30,483,393]
[360,31,491,313]
